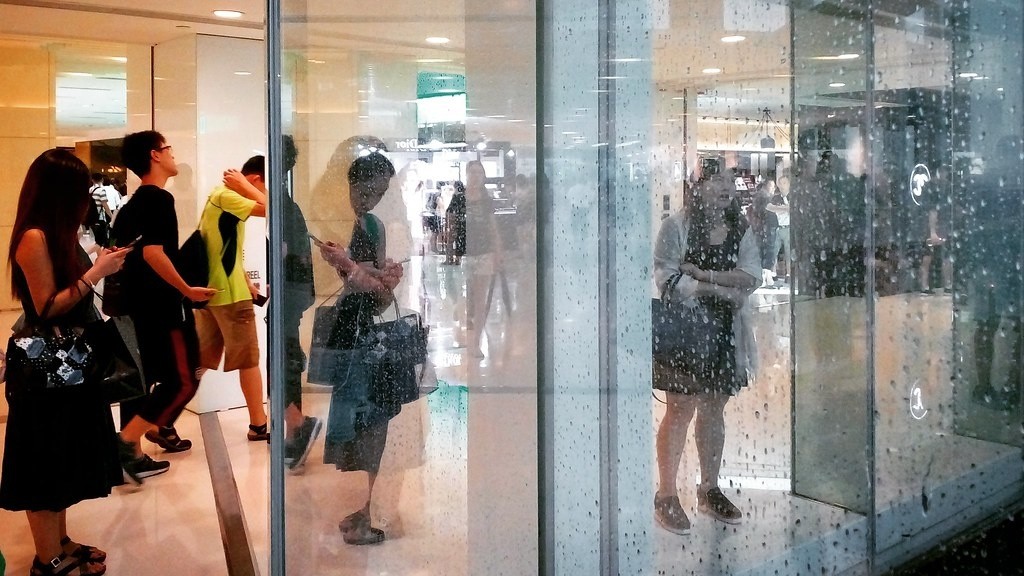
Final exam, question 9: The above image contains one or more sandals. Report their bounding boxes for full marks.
[339,509,370,533]
[29,554,107,576]
[61,536,106,561]
[343,519,404,546]
[247,422,269,440]
[146,424,192,452]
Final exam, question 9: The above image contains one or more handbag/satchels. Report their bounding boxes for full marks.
[7,283,150,417]
[654,273,718,392]
[308,281,352,383]
[362,280,439,411]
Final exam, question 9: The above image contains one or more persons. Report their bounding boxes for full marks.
[0,148,134,576]
[315,153,405,543]
[747,175,792,288]
[971,137,1024,409]
[919,168,952,296]
[280,134,322,470]
[146,154,266,451]
[85,130,217,487]
[467,161,536,358]
[426,180,465,265]
[655,151,764,535]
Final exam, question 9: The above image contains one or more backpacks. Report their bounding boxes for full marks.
[84,187,103,226]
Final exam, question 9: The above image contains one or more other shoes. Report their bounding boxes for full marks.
[122,468,143,487]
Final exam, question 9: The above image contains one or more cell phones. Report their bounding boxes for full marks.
[121,235,144,251]
[391,258,412,269]
[206,289,225,297]
[251,293,267,307]
[307,232,322,243]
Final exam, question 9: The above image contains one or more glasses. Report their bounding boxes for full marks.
[156,145,173,156]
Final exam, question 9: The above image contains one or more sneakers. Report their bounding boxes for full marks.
[654,492,690,534]
[283,416,323,471]
[696,486,743,524]
[126,455,170,477]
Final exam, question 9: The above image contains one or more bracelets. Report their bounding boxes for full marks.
[84,275,95,288]
[709,270,713,282]
[348,261,358,281]
